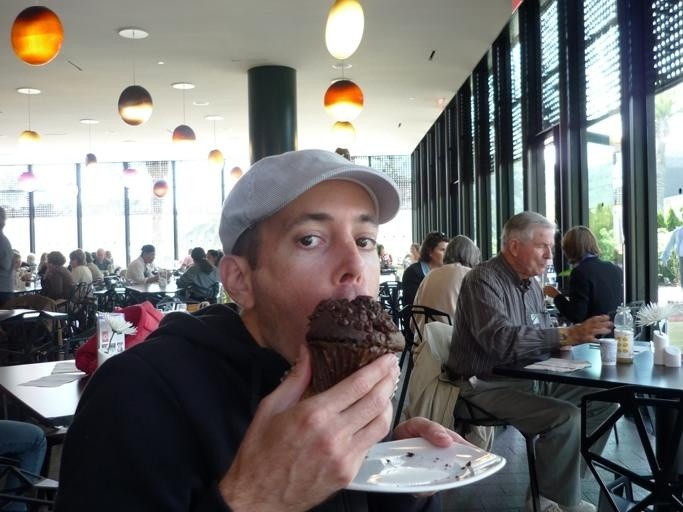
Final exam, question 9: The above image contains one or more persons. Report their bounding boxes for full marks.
[446,210,618,511]
[55,150,481,511]
[402,230,481,354]
[0,207,223,345]
[662,214,683,289]
[0,420,48,512]
[543,225,624,339]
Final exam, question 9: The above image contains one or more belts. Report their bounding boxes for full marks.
[453,376,484,382]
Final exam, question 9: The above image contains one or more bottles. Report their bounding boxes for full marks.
[615,306,634,364]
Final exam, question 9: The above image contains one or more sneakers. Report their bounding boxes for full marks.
[559,496,597,511]
[526,491,558,512]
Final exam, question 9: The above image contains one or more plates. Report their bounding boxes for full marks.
[341,434,508,495]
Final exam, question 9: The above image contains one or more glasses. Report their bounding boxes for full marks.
[430,231,444,243]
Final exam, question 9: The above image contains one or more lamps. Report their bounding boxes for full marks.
[11,1,63,190]
[326,0,364,163]
[85,28,243,199]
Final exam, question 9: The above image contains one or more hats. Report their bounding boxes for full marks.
[219,149,400,255]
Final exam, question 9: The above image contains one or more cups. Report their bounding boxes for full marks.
[545,282,558,304]
[599,338,618,366]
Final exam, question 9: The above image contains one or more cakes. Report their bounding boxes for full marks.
[306,295,405,393]
